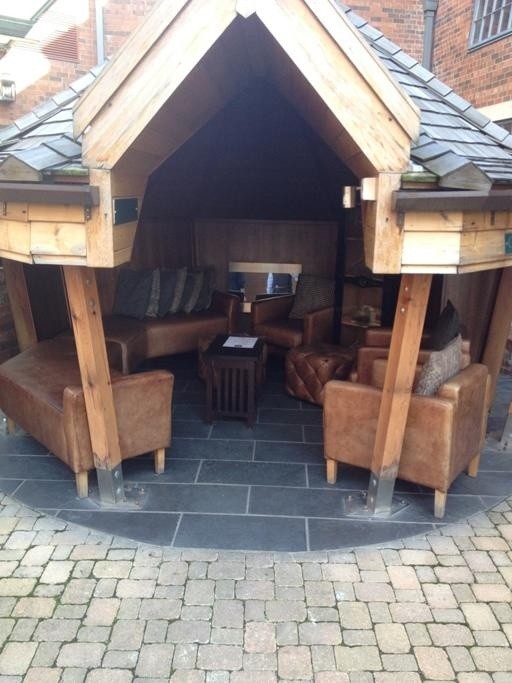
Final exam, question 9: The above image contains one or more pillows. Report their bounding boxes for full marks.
[426,300,459,351]
[415,333,463,397]
[112,262,215,319]
[288,273,334,319]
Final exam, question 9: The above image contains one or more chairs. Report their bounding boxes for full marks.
[320,358,492,518]
[356,327,471,388]
[251,293,334,356]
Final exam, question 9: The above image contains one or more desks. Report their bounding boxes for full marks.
[195,335,267,387]
[203,333,264,430]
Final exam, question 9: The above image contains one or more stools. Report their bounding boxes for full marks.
[284,341,356,406]
